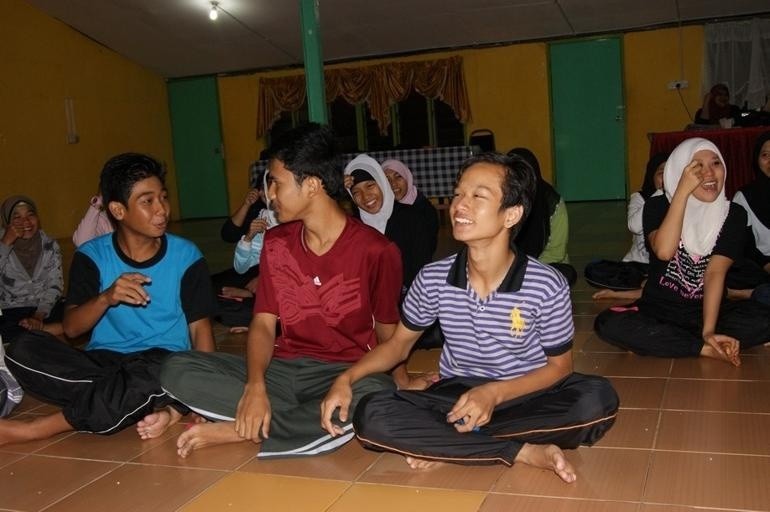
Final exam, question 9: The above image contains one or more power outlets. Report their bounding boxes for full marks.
[673,80,683,89]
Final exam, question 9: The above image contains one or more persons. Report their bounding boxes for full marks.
[695,83,741,125]
[503,147,577,289]
[159,122,433,462]
[584,152,667,301]
[593,137,770,367]
[3,193,70,346]
[341,152,446,349]
[0,154,213,448]
[208,171,282,339]
[72,190,114,250]
[319,152,619,484]
[729,134,769,288]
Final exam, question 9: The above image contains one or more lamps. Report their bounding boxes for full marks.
[210,2,218,21]
